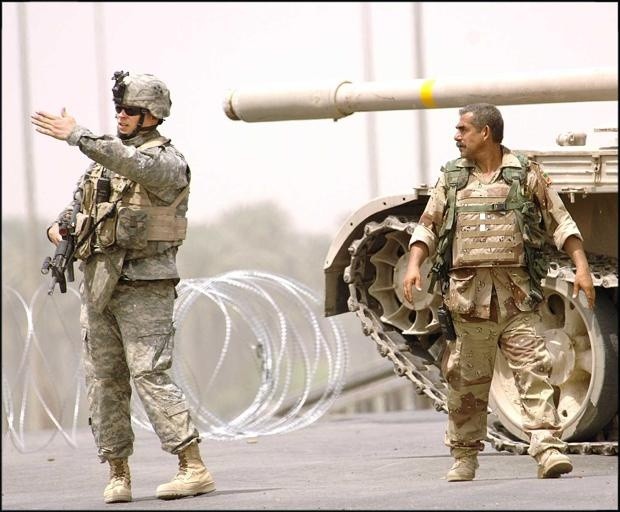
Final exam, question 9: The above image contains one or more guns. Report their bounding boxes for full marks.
[42,201,81,297]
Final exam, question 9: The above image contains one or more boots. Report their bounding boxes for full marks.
[156,440,217,497]
[103,456,132,502]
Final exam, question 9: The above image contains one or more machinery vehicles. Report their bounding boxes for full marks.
[219,67,620,460]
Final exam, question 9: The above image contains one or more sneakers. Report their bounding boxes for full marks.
[447,452,479,481]
[538,447,573,478]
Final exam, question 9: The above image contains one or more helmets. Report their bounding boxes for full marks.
[115,74,171,119]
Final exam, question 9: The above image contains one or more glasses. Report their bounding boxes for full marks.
[116,106,148,116]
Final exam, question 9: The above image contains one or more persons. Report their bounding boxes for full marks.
[31,73,216,504]
[402,101,598,483]
